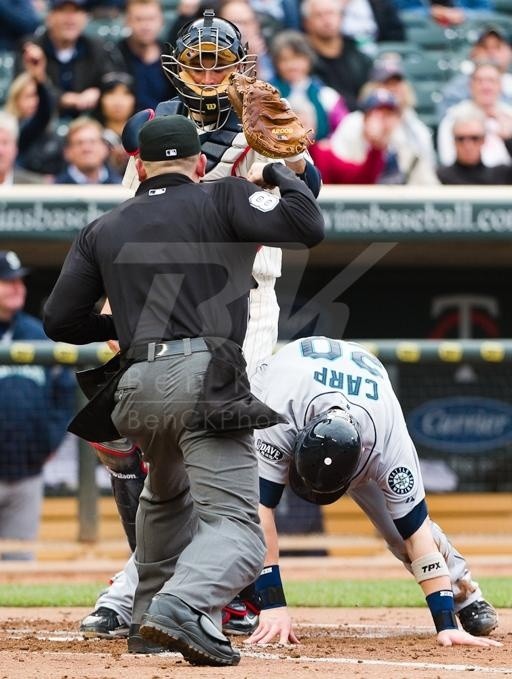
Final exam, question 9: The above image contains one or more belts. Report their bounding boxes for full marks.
[133,335,211,361]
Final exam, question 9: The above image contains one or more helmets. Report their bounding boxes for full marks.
[288,410,364,506]
[160,6,258,133]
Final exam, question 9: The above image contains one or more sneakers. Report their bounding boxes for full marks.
[221,595,261,637]
[80,606,131,640]
[127,622,169,654]
[455,583,499,637]
[140,594,240,668]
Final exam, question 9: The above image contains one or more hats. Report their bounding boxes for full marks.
[356,20,512,112]
[0,249,29,281]
[138,114,202,161]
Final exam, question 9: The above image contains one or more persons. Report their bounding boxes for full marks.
[1,2,511,188]
[77,334,510,652]
[2,245,76,564]
[42,114,328,668]
[78,12,324,642]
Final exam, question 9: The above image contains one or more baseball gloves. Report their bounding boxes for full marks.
[244,81,311,155]
